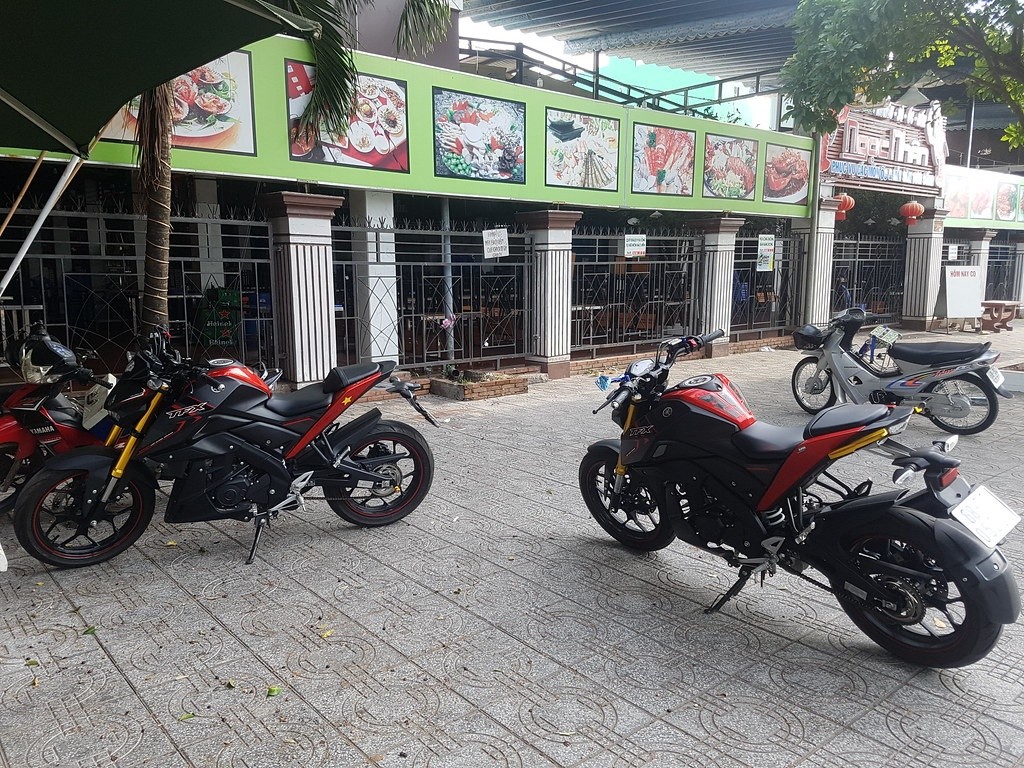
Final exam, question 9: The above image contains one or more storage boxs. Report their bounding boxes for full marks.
[871,300,885,314]
[201,287,241,348]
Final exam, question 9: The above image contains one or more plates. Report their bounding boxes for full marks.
[314,107,350,150]
[703,141,756,199]
[126,105,239,138]
[358,76,380,99]
[349,121,375,153]
[375,135,390,155]
[764,185,808,203]
[357,97,377,123]
[376,105,402,133]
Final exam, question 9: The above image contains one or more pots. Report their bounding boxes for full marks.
[289,114,315,159]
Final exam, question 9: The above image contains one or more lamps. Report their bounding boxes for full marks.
[650,210,663,219]
[889,217,902,226]
[536,62,545,88]
[642,88,648,108]
[863,218,876,225]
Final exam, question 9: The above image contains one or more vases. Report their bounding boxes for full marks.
[464,370,484,383]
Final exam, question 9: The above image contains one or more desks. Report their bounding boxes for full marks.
[981,300,1021,333]
[0,304,44,356]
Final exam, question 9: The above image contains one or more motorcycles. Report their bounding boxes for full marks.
[576,327,1021,669]
[0,320,284,514]
[790,309,1015,435]
[11,331,442,568]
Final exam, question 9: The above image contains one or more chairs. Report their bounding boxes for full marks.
[616,313,655,340]
[592,311,612,339]
